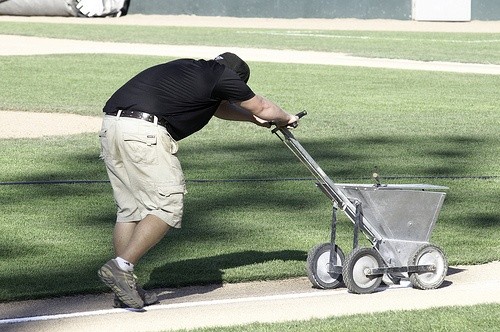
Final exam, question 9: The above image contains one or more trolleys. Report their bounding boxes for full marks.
[266,110,450,294]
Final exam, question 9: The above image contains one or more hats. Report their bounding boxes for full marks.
[215,52,250,83]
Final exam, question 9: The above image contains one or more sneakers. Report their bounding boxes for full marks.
[98,258,144,309]
[113,286,158,307]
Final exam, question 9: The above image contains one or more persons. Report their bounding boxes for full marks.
[98,51,299,309]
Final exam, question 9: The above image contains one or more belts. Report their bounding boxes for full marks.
[106,110,165,126]
